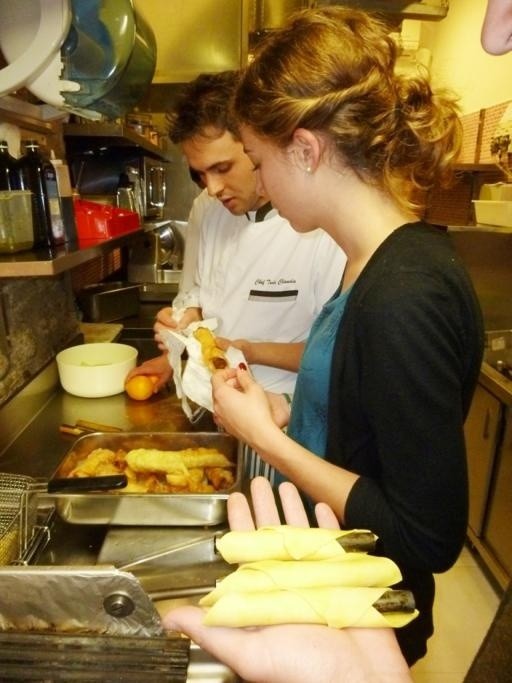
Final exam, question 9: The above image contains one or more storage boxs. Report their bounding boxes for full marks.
[470,200,512,228]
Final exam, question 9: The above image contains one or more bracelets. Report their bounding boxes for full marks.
[280,391,295,411]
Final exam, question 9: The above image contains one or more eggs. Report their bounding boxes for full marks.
[126,375,158,400]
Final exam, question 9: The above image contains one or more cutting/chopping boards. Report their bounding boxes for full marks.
[77,320,125,342]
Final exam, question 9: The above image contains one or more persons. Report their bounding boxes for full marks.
[153,470,420,683]
[151,68,351,495]
[124,166,205,397]
[209,0,489,668]
[480,0,512,60]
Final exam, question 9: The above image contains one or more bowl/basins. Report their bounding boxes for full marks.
[57,342,140,399]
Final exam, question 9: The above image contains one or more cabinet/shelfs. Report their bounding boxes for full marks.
[0,124,171,277]
[461,385,512,593]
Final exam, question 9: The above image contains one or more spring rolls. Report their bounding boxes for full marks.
[68,444,236,492]
[193,325,231,375]
[195,525,419,627]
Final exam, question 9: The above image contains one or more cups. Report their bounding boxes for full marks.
[1,192,36,254]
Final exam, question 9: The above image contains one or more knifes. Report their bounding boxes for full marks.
[33,475,128,492]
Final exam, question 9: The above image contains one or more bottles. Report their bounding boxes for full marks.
[116,167,144,225]
[1,139,78,250]
